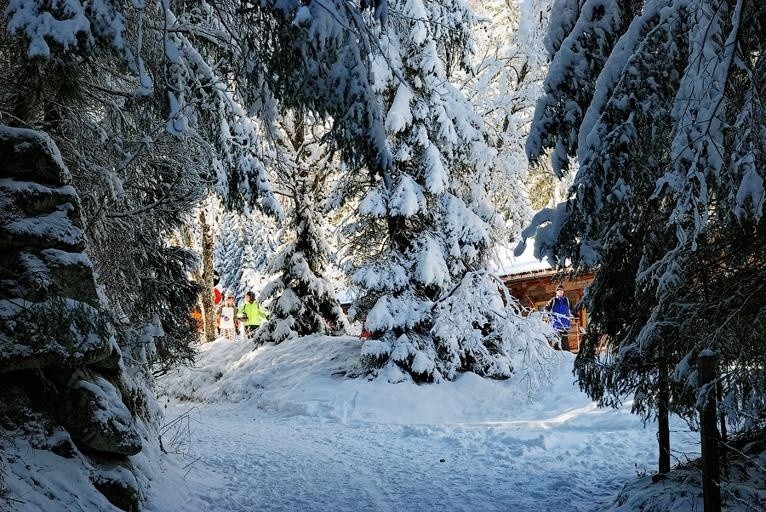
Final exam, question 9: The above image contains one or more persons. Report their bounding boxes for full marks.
[545,285,581,352]
[189,289,270,341]
